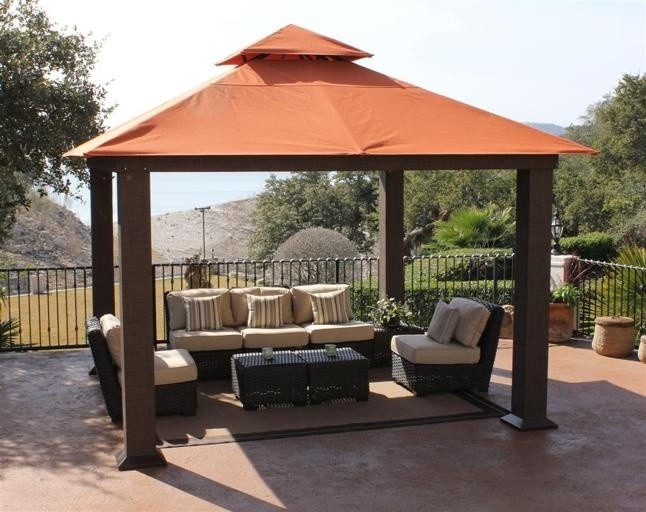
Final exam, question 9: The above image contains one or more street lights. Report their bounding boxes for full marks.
[193,204,210,260]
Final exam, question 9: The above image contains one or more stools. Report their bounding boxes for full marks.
[638,333,646,363]
[591,316,636,359]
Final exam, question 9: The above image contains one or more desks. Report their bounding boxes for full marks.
[369,321,425,369]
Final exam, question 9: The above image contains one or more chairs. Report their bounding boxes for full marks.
[82,313,201,417]
[386,296,505,398]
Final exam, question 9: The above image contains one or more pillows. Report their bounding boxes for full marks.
[304,288,351,326]
[179,292,227,333]
[427,298,460,346]
[242,290,287,329]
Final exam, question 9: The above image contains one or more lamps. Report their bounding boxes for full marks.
[551,209,566,255]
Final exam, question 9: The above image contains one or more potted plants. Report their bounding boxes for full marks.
[545,283,586,346]
[364,295,413,329]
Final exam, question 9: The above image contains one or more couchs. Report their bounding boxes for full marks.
[161,279,377,384]
